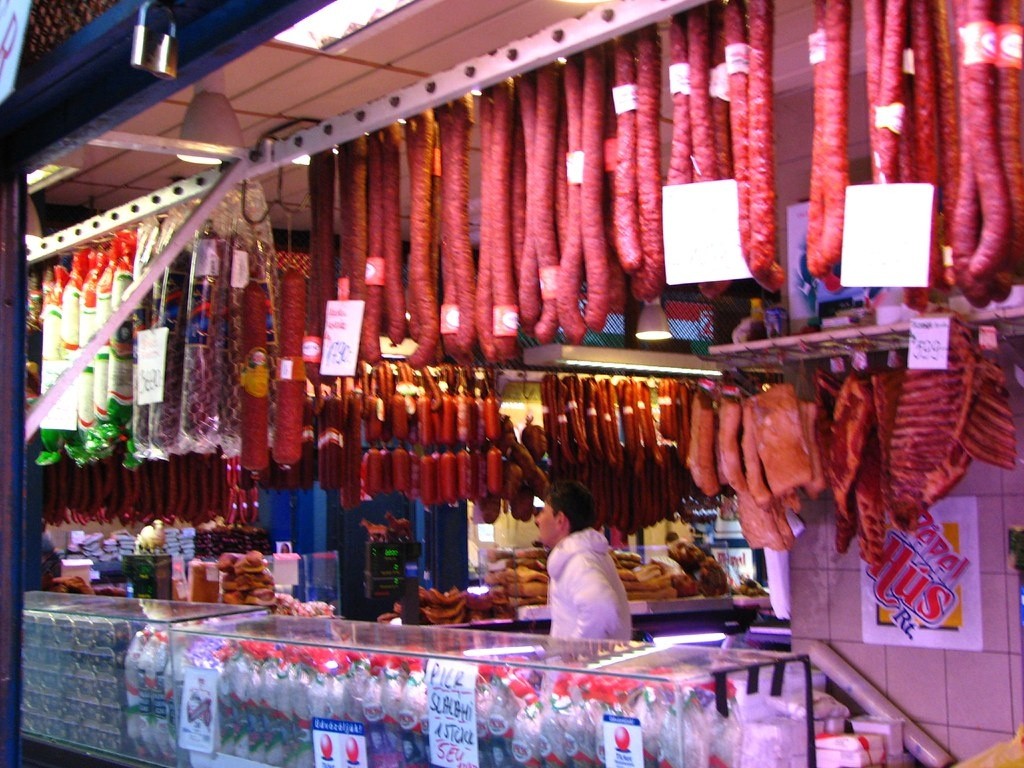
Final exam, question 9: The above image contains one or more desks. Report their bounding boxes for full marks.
[514,594,770,633]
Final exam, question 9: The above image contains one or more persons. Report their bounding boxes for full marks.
[535,481,631,640]
[664,522,715,559]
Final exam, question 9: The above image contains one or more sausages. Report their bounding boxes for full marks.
[39,1,1024,525]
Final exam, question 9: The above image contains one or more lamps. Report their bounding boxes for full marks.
[175,68,246,166]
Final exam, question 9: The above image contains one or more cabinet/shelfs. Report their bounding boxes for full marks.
[20,590,818,768]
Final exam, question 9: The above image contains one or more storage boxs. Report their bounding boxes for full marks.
[187,561,219,601]
[273,552,301,585]
[61,558,94,584]
[121,554,173,599]
